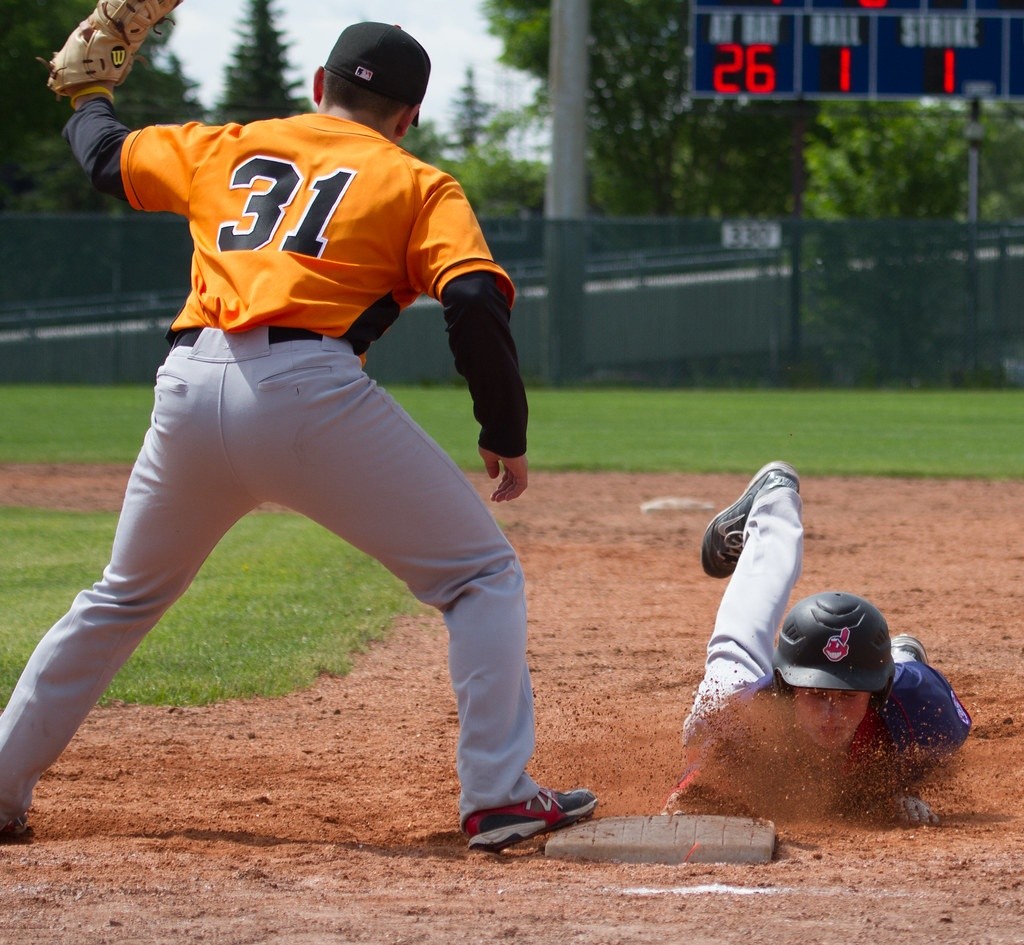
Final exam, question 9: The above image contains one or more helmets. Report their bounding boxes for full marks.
[772,592,894,705]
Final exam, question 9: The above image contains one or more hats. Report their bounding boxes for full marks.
[324,23,430,128]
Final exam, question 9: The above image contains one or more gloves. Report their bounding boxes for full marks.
[887,794,939,827]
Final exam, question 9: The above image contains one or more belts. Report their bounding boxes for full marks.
[173,327,322,347]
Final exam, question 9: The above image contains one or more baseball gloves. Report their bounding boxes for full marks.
[40,0,179,108]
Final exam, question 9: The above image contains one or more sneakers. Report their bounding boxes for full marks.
[464,788,596,852]
[891,634,928,665]
[701,460,800,578]
[0,814,35,839]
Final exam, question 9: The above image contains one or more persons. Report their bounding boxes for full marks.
[663,460,973,828]
[1,0,596,853]
